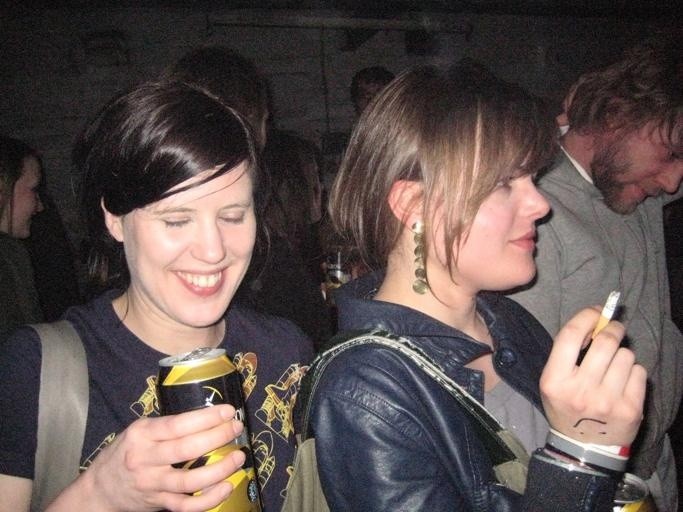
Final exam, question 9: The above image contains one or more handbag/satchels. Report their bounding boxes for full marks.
[279,328,531,512]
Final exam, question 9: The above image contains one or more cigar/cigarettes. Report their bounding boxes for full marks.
[590,287,622,340]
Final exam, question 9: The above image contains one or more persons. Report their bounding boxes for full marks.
[501,30,682,512]
[1,136,44,343]
[1,79,316,512]
[300,62,648,511]
[350,70,397,115]
[172,45,292,318]
[263,128,337,345]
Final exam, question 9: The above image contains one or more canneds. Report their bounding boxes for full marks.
[326,244,352,291]
[612,472,657,512]
[153,348,263,512]
